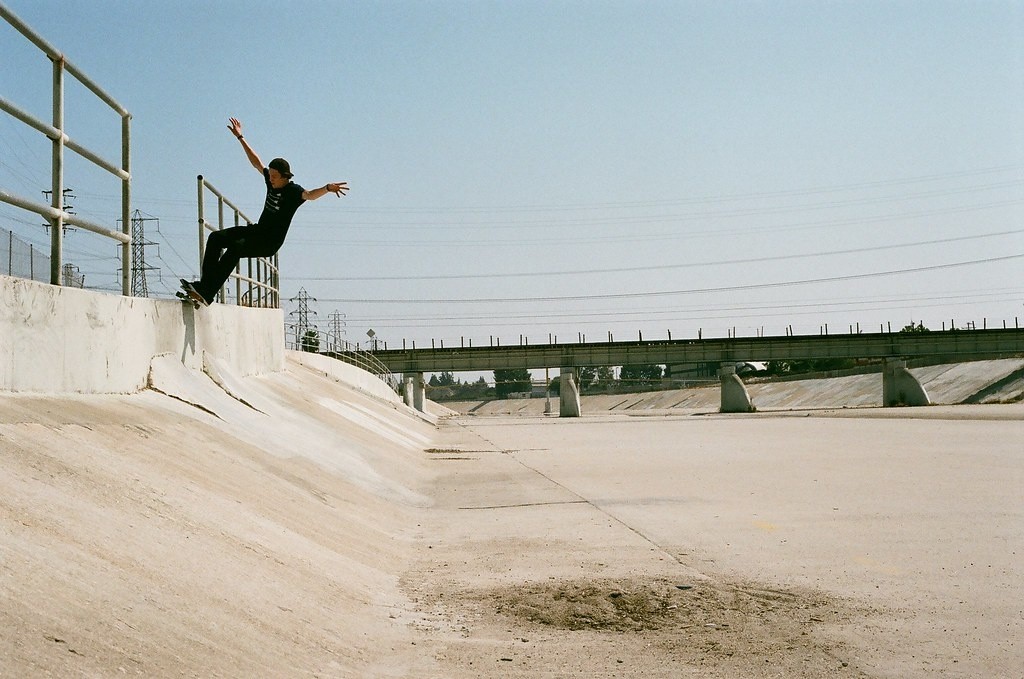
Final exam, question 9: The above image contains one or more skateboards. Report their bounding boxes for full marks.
[175,278,209,311]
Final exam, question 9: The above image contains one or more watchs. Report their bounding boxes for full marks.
[237,134,244,140]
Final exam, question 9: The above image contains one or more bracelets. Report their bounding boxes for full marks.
[325,184,330,193]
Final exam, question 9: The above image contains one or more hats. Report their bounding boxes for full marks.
[269,158,295,176]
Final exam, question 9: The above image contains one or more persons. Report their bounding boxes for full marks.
[180,117,350,307]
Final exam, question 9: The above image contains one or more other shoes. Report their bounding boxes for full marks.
[180,279,214,307]
